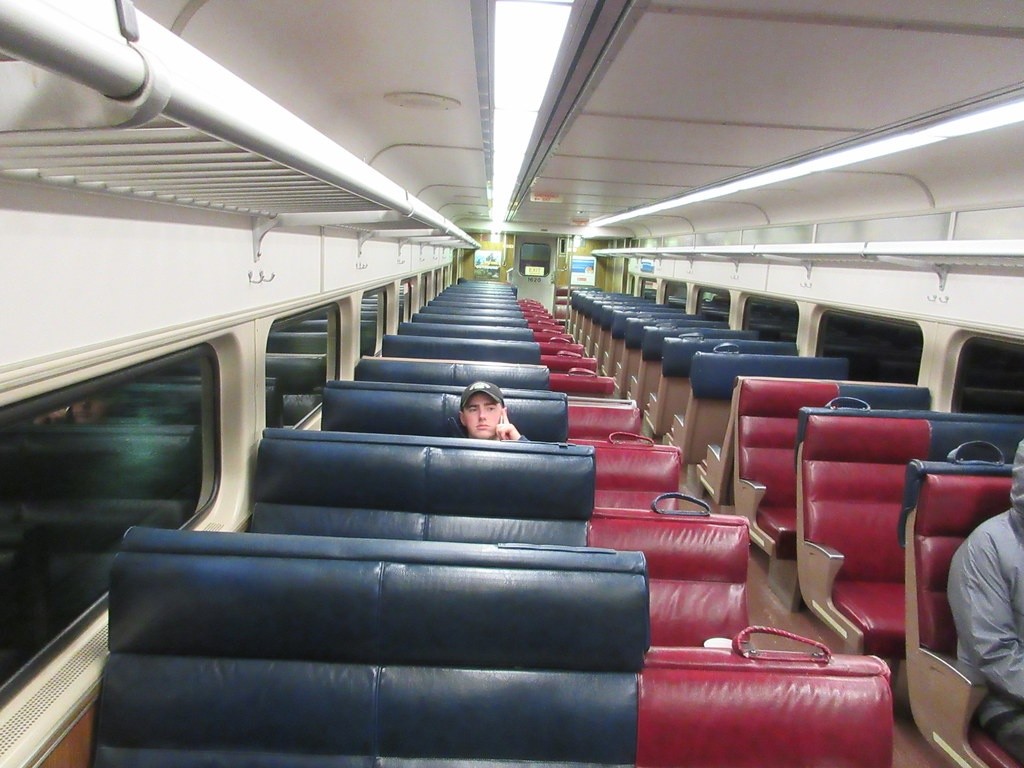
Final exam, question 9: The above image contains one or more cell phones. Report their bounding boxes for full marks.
[499,416,504,424]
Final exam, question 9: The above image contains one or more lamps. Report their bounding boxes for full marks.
[0,0,1024,279]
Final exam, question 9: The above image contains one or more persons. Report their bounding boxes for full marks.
[459,380,527,441]
[33,389,118,424]
[946,438,1024,768]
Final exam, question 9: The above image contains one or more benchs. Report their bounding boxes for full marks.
[91,281,1024,768]
[0,283,423,685]
